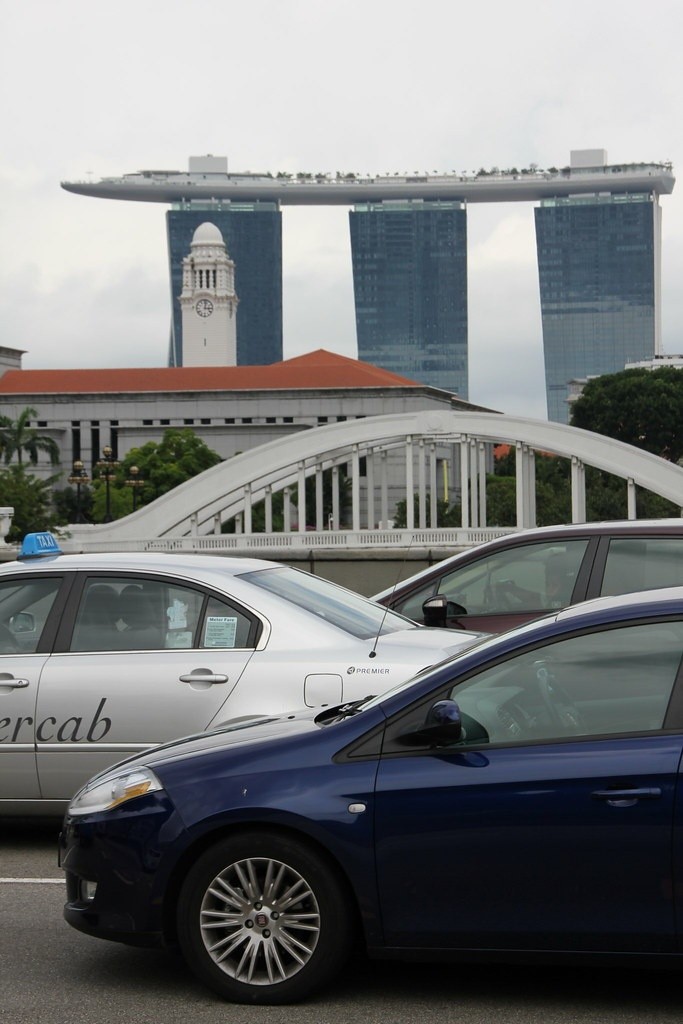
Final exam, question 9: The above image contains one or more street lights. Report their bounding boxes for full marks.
[95,445,120,523]
[68,461,90,523]
[124,466,144,512]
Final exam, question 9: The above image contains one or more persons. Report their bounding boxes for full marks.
[495,552,569,609]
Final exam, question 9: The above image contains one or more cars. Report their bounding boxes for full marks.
[59,585,683,1005]
[0,552,489,814]
[367,518,683,635]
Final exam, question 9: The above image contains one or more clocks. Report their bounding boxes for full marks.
[198,300,214,316]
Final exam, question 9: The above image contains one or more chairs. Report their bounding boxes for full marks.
[115,585,164,649]
